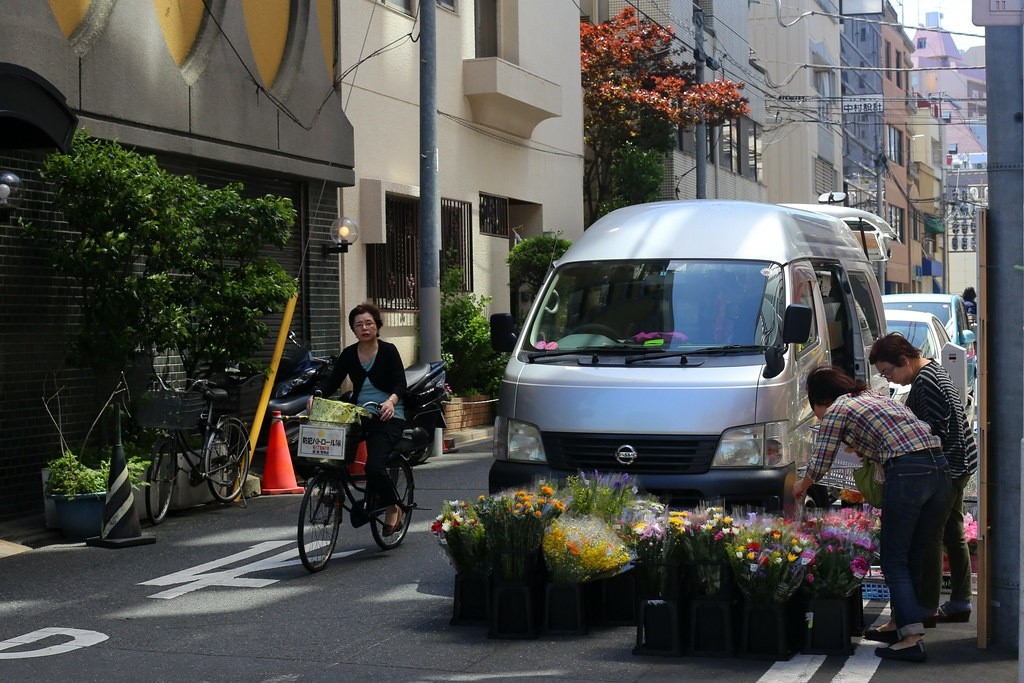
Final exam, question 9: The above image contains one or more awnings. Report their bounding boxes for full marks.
[917,92,930,109]
[922,257,943,277]
[933,279,943,294]
[924,217,944,234]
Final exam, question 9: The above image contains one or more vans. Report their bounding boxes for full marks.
[488,197,901,520]
[879,292,979,407]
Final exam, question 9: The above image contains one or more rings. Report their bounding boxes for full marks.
[386,409,392,415]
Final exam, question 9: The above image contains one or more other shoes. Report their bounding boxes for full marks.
[382,506,403,537]
[863,629,900,645]
[320,495,337,505]
[875,640,928,663]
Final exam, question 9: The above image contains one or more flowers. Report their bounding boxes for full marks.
[430,468,882,605]
[956,502,983,553]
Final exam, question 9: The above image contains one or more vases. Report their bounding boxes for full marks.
[802,598,851,656]
[739,607,789,660]
[688,598,733,659]
[849,587,864,637]
[449,567,492,626]
[631,598,684,656]
[487,581,540,638]
[543,581,583,637]
[600,579,637,627]
[941,543,981,572]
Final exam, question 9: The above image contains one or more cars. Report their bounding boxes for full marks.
[883,309,954,410]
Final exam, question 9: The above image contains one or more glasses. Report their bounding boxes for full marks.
[353,321,376,327]
[879,363,899,382]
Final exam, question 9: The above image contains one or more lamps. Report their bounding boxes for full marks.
[322,217,359,258]
[0,171,26,221]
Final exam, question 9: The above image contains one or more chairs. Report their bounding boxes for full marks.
[587,265,643,340]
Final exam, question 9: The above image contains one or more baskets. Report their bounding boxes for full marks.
[306,420,356,465]
[138,389,205,432]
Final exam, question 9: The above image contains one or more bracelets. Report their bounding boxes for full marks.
[388,399,395,406]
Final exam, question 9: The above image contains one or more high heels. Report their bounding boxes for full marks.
[936,601,973,623]
[922,615,939,628]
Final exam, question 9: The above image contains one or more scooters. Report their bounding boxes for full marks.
[217,330,453,483]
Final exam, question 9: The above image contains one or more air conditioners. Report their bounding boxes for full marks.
[924,241,933,256]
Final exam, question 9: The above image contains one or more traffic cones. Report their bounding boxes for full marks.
[260,412,305,496]
[84,393,158,550]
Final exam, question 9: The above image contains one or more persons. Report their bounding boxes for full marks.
[961,287,978,332]
[869,331,979,629]
[793,365,949,662]
[307,303,407,537]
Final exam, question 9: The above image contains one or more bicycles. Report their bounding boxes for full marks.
[297,388,433,578]
[137,372,254,525]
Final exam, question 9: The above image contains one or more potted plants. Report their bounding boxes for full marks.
[410,243,512,434]
[126,424,229,512]
[41,392,189,531]
[45,448,152,541]
